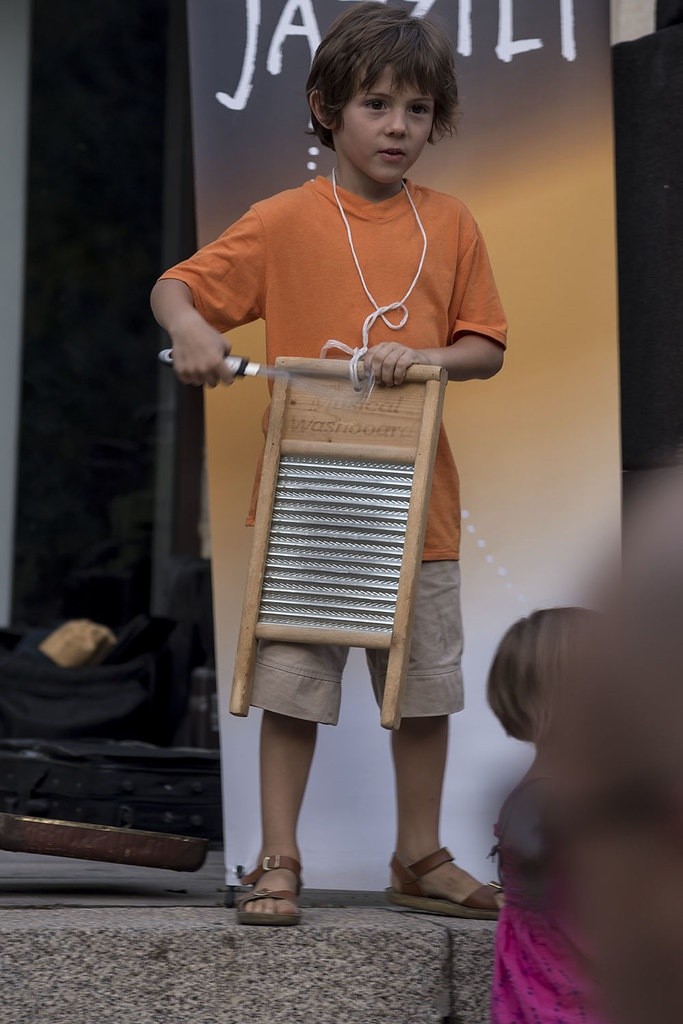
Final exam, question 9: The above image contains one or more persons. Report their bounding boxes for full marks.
[484,603,608,1022]
[148,1,510,926]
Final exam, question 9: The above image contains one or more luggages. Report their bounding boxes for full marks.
[0,736,222,846]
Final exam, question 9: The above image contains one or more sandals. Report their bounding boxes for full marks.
[383,846,502,920]
[235,855,304,924]
